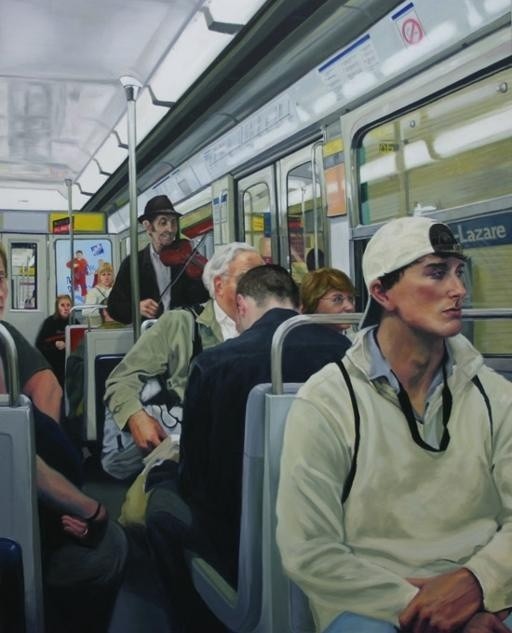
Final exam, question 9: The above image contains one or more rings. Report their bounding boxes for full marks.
[82,528,89,537]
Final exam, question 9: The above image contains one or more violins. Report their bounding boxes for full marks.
[159,239,208,277]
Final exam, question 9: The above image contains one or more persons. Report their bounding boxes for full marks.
[0,241,66,426]
[144,262,351,633]
[82,263,115,325]
[300,267,360,343]
[0,348,128,632]
[106,194,210,326]
[103,241,266,595]
[66,250,90,296]
[274,215,512,632]
[34,293,84,388]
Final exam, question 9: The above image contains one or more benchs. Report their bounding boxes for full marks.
[0,301,512,633]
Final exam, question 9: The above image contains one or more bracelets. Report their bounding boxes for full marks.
[84,500,102,523]
